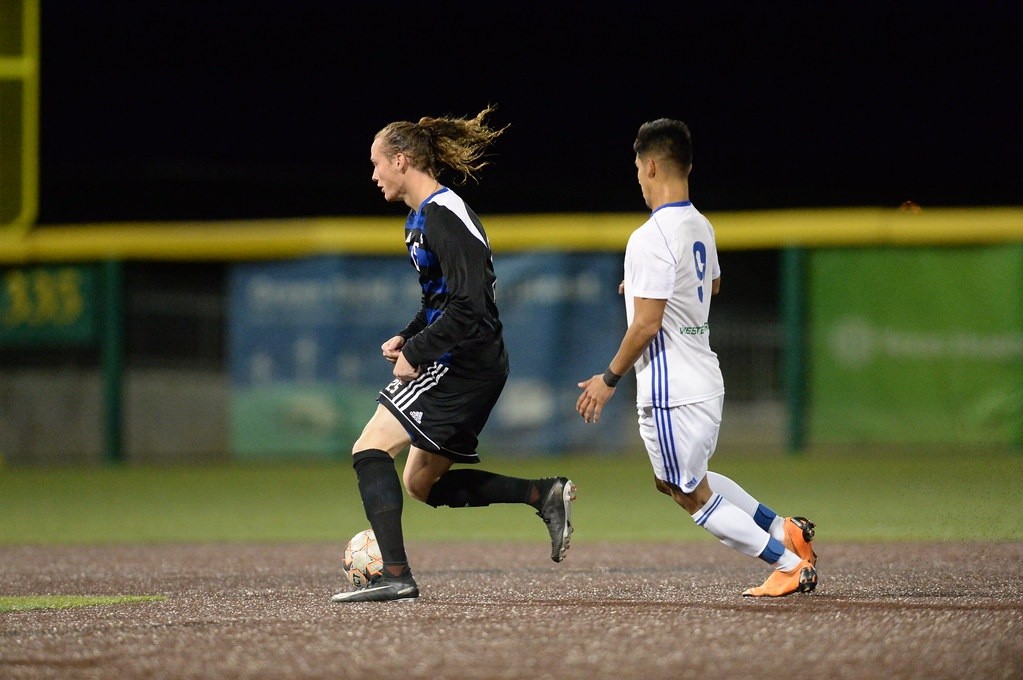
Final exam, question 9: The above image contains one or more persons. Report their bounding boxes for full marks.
[331,109,577,603]
[576,119,819,597]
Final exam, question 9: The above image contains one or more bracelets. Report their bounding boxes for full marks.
[603,367,622,387]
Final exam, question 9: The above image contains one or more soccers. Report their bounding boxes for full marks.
[342,529,384,589]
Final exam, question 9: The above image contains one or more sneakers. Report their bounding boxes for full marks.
[535,476,577,563]
[783,516,817,568]
[742,560,817,596]
[332,566,419,602]
